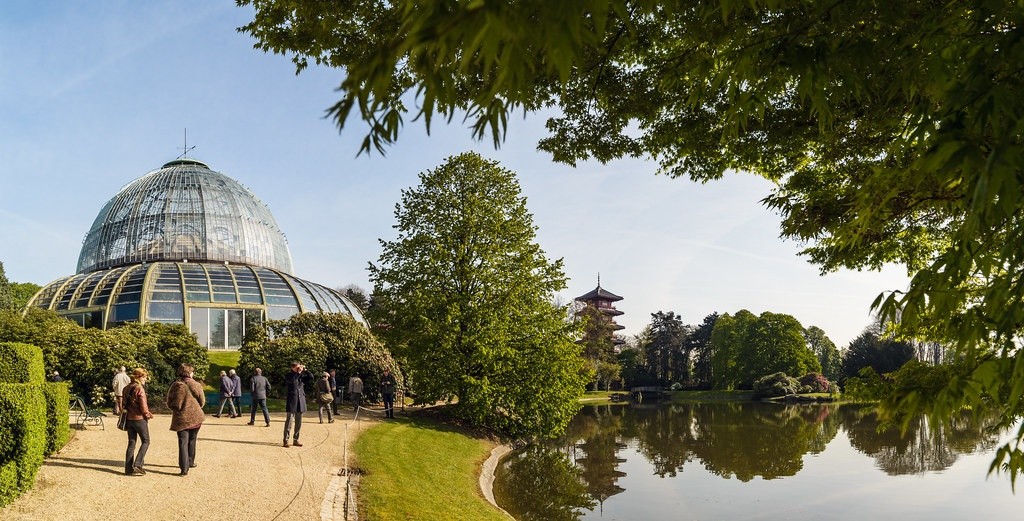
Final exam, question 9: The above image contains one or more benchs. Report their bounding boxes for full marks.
[74,397,107,430]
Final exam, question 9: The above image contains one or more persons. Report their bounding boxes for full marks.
[282,362,313,447]
[315,372,335,423]
[328,369,340,415]
[122,368,153,477]
[348,372,364,412]
[167,364,205,475]
[212,370,236,418]
[247,368,272,426]
[225,369,242,417]
[112,366,131,415]
[379,369,396,419]
[51,371,62,382]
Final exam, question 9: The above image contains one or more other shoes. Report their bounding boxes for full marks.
[125,471,143,476]
[231,416,235,418]
[384,416,389,418]
[189,463,197,467]
[225,415,232,417]
[212,415,220,418]
[133,466,146,474]
[180,468,188,475]
[266,424,270,427]
[320,421,323,423]
[333,412,340,415]
[248,422,254,425]
[329,419,334,423]
[283,441,289,447]
[237,414,241,417]
[293,440,302,446]
[390,416,397,419]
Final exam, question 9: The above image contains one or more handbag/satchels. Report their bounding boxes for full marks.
[320,392,333,402]
[117,408,127,431]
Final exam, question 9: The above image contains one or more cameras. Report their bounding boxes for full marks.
[303,366,308,370]
